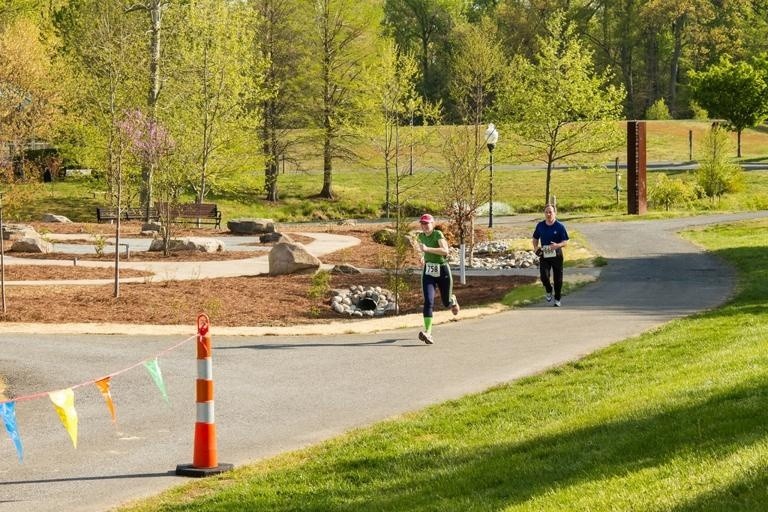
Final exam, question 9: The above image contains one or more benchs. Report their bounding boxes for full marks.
[155,203,221,229]
[98,208,160,223]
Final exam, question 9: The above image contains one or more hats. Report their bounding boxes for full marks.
[419,214,434,223]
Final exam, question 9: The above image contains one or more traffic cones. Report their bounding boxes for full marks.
[172,311,235,478]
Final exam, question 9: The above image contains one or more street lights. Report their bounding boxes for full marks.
[485,123,500,229]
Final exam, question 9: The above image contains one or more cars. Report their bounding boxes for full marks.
[14,145,105,181]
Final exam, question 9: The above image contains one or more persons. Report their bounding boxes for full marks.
[534,205,569,307]
[416,214,460,344]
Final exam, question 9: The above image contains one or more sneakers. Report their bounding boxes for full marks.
[419,331,433,344]
[451,295,459,315]
[546,292,552,302]
[554,300,561,307]
